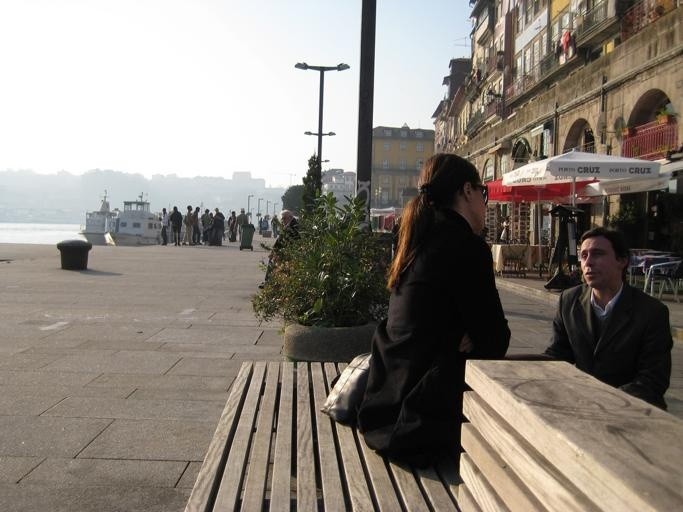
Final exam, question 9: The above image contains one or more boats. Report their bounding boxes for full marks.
[76,188,233,247]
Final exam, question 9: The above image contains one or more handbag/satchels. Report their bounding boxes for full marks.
[318,349,373,430]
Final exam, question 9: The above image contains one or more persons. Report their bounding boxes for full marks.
[159,204,279,247]
[544,227,672,416]
[357,151,553,463]
[258,211,297,290]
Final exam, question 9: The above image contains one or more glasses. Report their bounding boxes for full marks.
[472,183,489,206]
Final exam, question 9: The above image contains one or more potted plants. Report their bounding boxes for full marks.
[245,192,399,361]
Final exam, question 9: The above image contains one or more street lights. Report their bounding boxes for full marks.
[246,194,279,224]
[292,62,349,189]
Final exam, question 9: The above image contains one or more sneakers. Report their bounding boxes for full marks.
[161,240,209,247]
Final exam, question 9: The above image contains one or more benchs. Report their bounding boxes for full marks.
[184,356,464,511]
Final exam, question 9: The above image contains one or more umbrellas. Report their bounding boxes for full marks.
[484,178,600,260]
[502,146,661,221]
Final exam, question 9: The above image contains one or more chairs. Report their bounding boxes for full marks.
[489,231,683,304]
